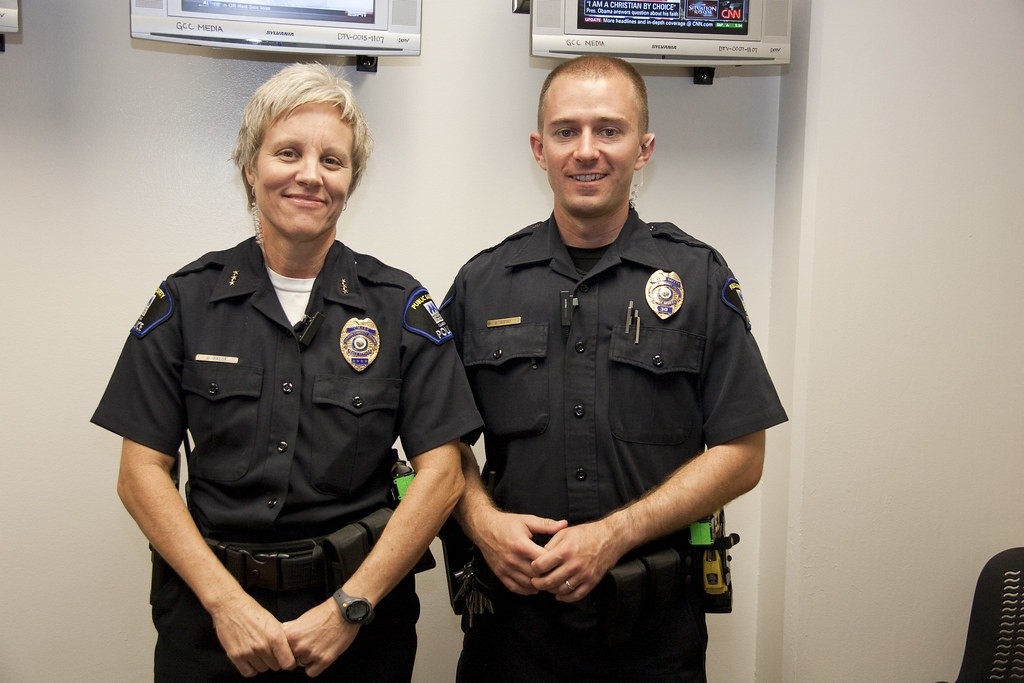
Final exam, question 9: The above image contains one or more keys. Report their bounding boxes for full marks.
[452,568,494,629]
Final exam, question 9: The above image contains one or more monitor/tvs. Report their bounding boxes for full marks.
[530,0,792,65]
[130,0,422,57]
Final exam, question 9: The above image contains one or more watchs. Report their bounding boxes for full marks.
[333,588,375,625]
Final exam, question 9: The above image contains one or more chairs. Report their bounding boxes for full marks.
[955,546,1024,683]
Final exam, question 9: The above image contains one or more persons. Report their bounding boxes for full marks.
[89,64,485,683]
[437,53,789,683]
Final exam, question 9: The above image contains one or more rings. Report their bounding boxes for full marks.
[565,580,575,590]
[298,657,305,667]
